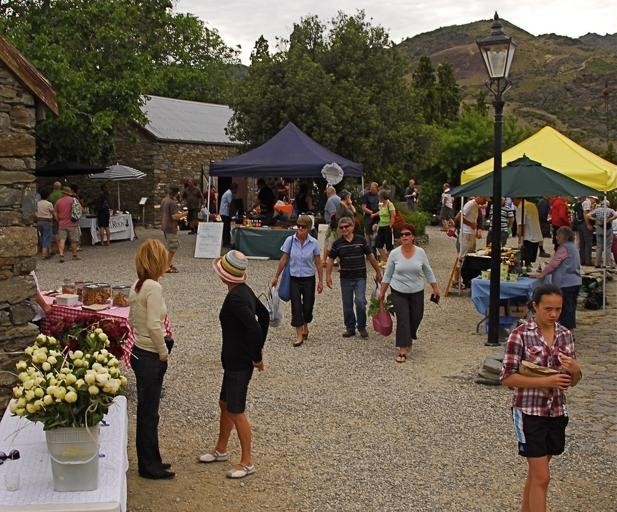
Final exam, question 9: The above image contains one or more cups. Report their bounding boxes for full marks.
[499,264,508,281]
[481,270,493,281]
[509,274,520,283]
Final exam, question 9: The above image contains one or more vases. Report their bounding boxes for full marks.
[44,426,98,489]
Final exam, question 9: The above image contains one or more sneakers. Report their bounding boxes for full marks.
[343,330,355,337]
[59,256,64,263]
[226,464,256,479]
[154,472,175,480]
[72,256,82,260]
[159,463,171,469]
[200,450,228,462]
[359,327,368,338]
[452,280,466,289]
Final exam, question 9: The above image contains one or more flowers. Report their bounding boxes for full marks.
[9,325,128,428]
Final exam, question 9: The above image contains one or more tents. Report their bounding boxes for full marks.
[205,121,365,220]
[451,125,617,312]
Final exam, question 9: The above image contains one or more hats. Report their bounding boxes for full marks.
[589,196,599,200]
[601,199,610,206]
[168,184,181,192]
[212,250,249,283]
[59,186,74,195]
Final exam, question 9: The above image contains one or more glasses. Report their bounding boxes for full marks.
[339,225,351,229]
[399,232,413,236]
[297,224,307,229]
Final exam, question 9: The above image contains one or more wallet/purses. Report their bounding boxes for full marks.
[430,294,439,304]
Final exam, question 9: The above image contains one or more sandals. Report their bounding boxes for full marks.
[302,329,308,340]
[293,337,303,347]
[396,354,406,363]
[164,269,179,273]
[170,264,177,270]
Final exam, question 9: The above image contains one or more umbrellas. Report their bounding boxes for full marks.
[89,162,147,213]
[35,152,109,178]
[444,154,607,318]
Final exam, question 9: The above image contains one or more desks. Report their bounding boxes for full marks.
[472,272,550,320]
[37,288,172,369]
[461,246,521,288]
[1,392,132,512]
[237,226,315,260]
[77,214,133,246]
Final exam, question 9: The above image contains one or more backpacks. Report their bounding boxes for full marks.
[571,201,585,225]
[71,198,81,222]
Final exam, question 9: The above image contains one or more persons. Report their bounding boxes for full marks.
[475,201,488,238]
[128,239,176,480]
[403,179,418,212]
[361,181,381,260]
[511,197,544,268]
[35,189,53,260]
[456,196,491,266]
[327,216,382,339]
[372,191,397,262]
[384,184,397,200]
[501,284,582,512]
[47,181,64,253]
[588,200,616,268]
[550,197,570,252]
[324,184,340,222]
[610,209,617,266]
[184,179,192,198]
[160,185,188,273]
[68,191,83,251]
[272,214,323,348]
[220,183,239,248]
[295,183,318,211]
[30,270,51,329]
[379,224,441,362]
[439,183,455,231]
[342,189,357,217]
[196,250,269,479]
[54,186,81,262]
[189,180,203,234]
[35,181,42,200]
[97,184,111,246]
[204,184,217,214]
[579,195,599,266]
[249,178,275,225]
[272,176,291,203]
[487,197,515,246]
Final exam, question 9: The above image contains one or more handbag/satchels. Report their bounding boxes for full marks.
[278,235,294,302]
[372,275,387,305]
[71,198,82,222]
[388,201,405,229]
[518,360,559,398]
